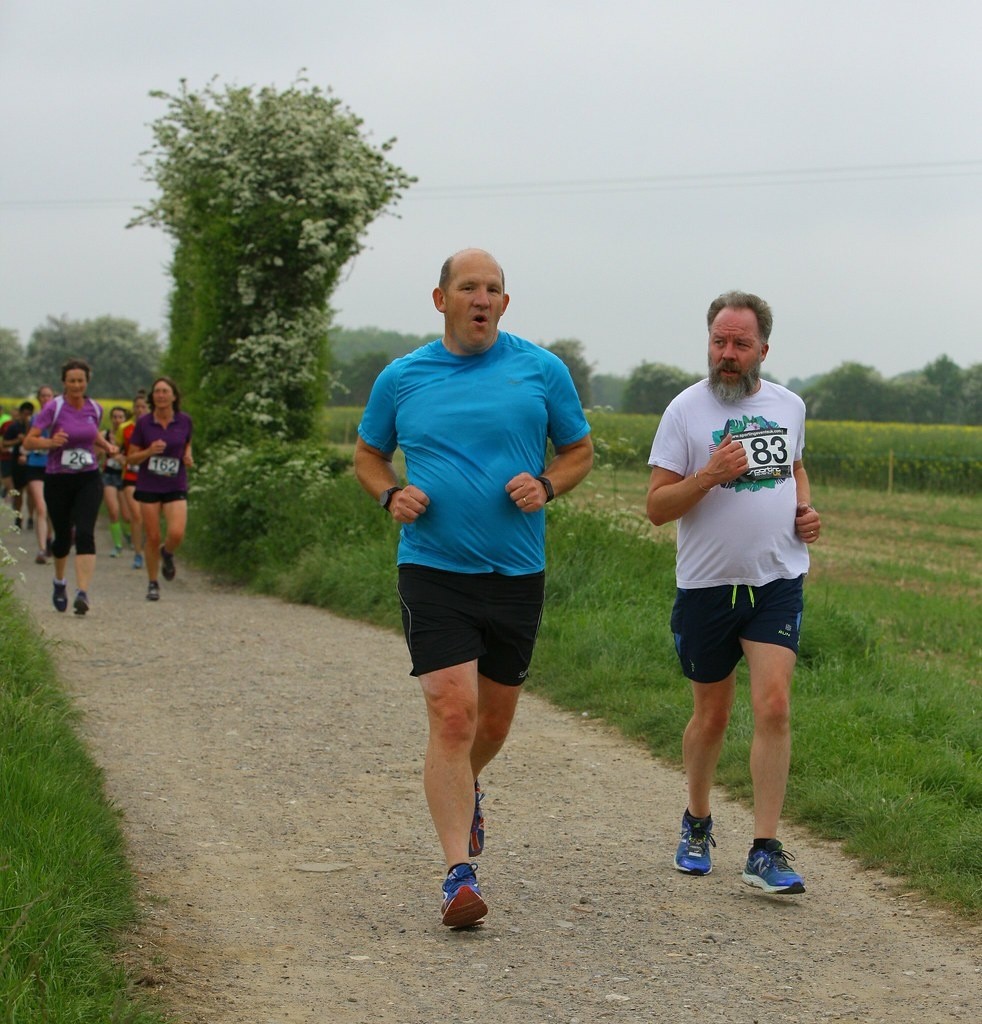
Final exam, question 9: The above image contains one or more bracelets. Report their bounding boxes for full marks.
[695,471,709,492]
[809,506,814,509]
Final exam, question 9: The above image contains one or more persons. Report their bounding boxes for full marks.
[0,385,52,563]
[22,360,121,615]
[647,293,821,895]
[97,407,134,559]
[128,379,194,599]
[354,249,594,932]
[115,389,149,569]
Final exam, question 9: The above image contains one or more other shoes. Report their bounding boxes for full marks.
[133,556,142,568]
[110,547,124,557]
[35,555,46,564]
[46,539,53,557]
[27,519,33,531]
[124,535,133,549]
[14,517,23,531]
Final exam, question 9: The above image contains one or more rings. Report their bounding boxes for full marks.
[811,531,814,535]
[524,498,528,505]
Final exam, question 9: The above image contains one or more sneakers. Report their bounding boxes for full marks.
[673,809,716,875]
[441,862,487,929]
[468,780,485,856]
[52,581,67,612]
[160,545,175,581]
[741,839,804,894]
[147,584,160,600]
[73,597,90,615]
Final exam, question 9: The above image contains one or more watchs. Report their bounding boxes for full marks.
[536,477,554,503]
[380,487,402,511]
